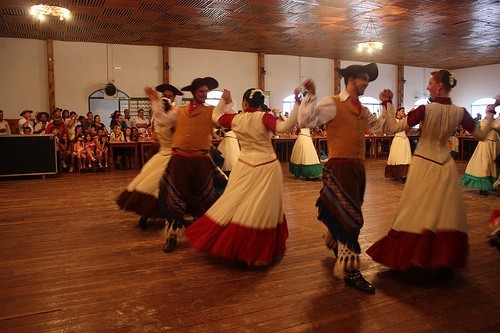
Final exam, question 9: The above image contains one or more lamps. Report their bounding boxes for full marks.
[28,3,71,23]
[355,16,383,53]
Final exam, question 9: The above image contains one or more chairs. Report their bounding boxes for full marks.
[69,141,110,172]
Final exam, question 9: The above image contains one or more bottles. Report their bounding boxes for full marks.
[28,114,34,118]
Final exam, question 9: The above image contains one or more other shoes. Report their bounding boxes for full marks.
[417,267,451,287]
[236,260,246,269]
[344,271,376,293]
[62,157,109,173]
[163,238,177,252]
[399,176,406,183]
[490,236,500,246]
[307,176,317,181]
[479,190,488,196]
[122,161,126,169]
[115,163,119,170]
[138,215,148,227]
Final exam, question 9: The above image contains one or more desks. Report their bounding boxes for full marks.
[107,135,476,173]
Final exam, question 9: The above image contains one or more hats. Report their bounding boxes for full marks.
[70,111,76,115]
[182,76,218,91]
[77,134,83,137]
[20,110,33,116]
[79,116,85,120]
[52,107,62,114]
[37,112,49,121]
[132,126,138,132]
[336,62,379,82]
[54,115,62,120]
[155,83,184,96]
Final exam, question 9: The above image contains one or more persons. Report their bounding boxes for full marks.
[297,62,393,292]
[267,108,328,162]
[144,77,237,253]
[18,110,50,135]
[384,107,412,184]
[289,128,323,181]
[109,109,160,170]
[365,113,419,157]
[45,108,109,172]
[448,94,500,246]
[365,70,497,279]
[212,110,243,176]
[182,87,302,269]
[0,109,11,135]
[114,84,229,230]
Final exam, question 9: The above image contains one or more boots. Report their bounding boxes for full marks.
[129,159,135,169]
